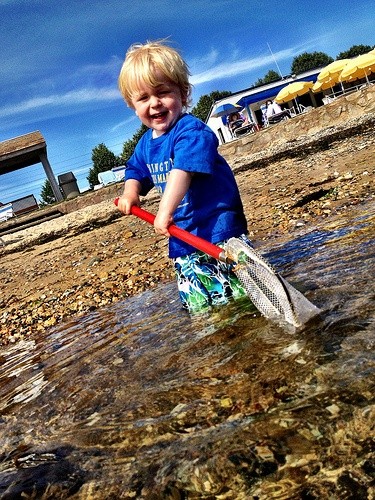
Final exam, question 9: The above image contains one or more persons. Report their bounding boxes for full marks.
[117,41,252,314]
[260,100,282,118]
[228,112,250,132]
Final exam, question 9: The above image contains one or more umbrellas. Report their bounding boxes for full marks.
[210,49,375,118]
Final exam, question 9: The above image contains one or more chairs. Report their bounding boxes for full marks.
[98,165,126,187]
[260,100,292,127]
[225,112,260,140]
